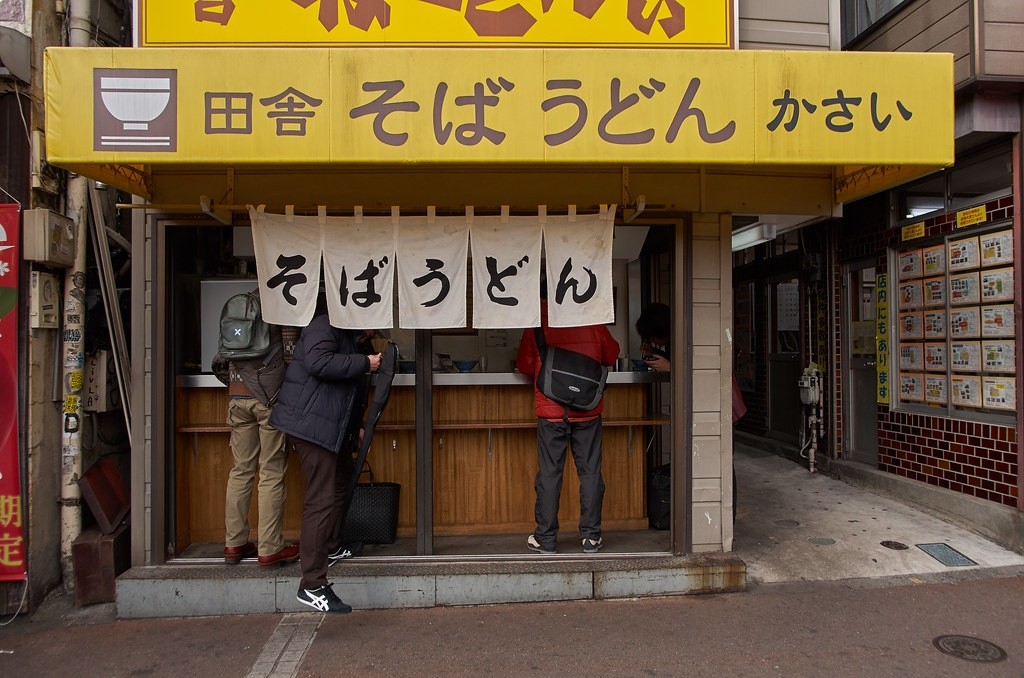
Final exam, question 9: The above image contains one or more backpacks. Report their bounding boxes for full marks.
[218,292,271,358]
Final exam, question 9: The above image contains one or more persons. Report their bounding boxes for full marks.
[268,312,382,614]
[636,303,748,525]
[212,288,300,571]
[517,278,620,553]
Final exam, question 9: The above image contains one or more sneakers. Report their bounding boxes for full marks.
[327,542,364,567]
[527,534,557,554]
[582,537,603,553]
[296,583,352,615]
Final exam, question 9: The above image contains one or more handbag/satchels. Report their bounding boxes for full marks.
[536,347,609,411]
[647,463,671,530]
[339,460,401,544]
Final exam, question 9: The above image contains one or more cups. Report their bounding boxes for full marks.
[613,358,623,372]
[479,355,488,372]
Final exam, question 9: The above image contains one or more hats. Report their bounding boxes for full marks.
[375,330,391,340]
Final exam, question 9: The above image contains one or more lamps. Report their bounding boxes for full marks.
[731,223,777,252]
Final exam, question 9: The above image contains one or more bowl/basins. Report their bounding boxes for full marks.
[453,360,478,372]
[631,360,649,370]
[399,360,416,373]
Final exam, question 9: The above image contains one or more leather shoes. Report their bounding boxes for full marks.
[258,542,300,566]
[223,542,258,564]
